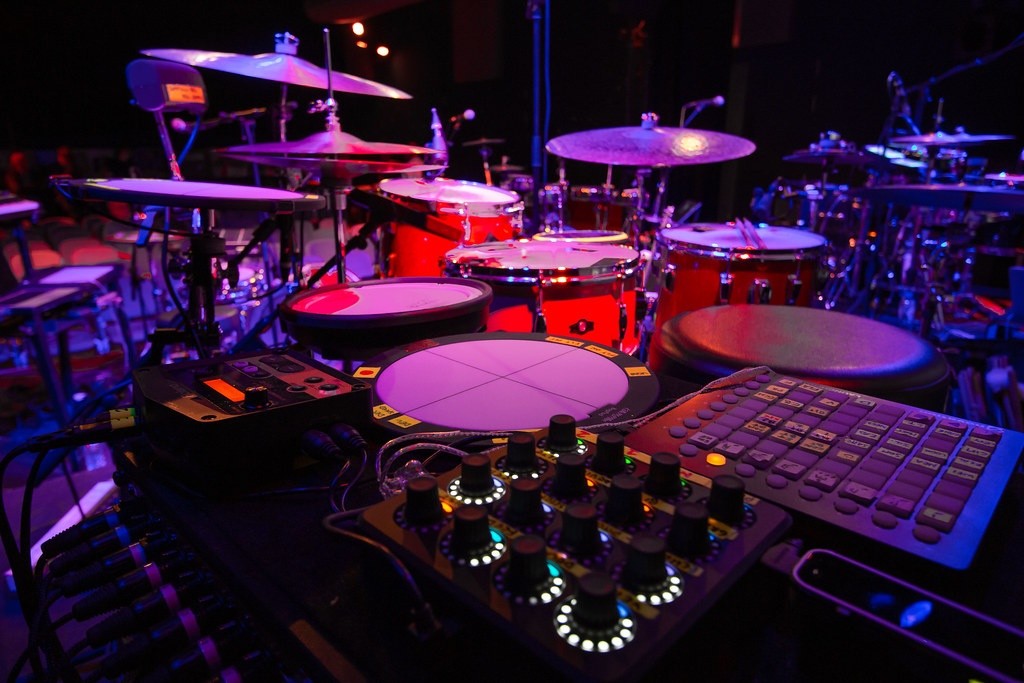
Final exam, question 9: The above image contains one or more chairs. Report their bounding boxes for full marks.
[0,198,137,425]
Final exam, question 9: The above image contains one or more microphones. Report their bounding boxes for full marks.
[893,75,911,116]
[684,97,725,109]
[450,110,476,123]
[431,110,448,164]
[173,119,195,134]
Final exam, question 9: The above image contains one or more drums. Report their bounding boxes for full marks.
[976,173,1024,256]
[769,179,867,256]
[539,182,650,236]
[278,274,494,360]
[443,237,646,355]
[647,222,831,374]
[657,302,954,405]
[350,332,660,441]
[53,176,327,214]
[370,174,524,281]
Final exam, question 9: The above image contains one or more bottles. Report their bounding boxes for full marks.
[74,393,107,471]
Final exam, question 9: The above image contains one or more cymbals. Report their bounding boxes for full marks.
[781,149,875,167]
[462,139,505,145]
[211,131,449,177]
[489,166,525,171]
[869,185,1024,212]
[865,145,905,159]
[140,44,411,101]
[547,126,755,166]
[890,134,1014,147]
[890,158,930,168]
